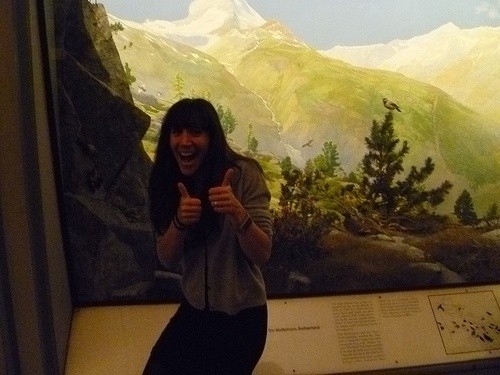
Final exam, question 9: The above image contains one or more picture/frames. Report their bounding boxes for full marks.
[35,0,500,310]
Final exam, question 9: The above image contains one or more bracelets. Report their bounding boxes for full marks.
[172,216,186,231]
[175,214,191,228]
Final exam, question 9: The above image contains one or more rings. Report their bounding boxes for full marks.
[215,201,218,208]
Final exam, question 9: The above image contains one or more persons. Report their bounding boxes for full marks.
[143,98,274,375]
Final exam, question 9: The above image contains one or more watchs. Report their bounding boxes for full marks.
[237,212,253,233]
